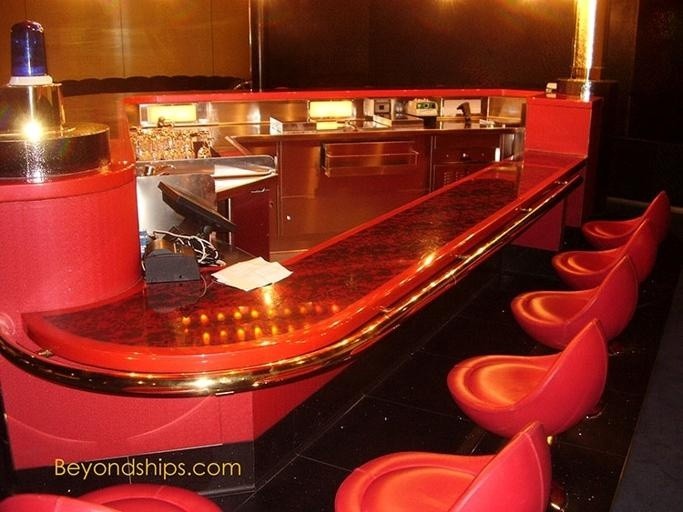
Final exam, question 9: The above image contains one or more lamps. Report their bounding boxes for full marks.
[7,16,53,118]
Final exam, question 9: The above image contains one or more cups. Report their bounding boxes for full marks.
[127,126,212,161]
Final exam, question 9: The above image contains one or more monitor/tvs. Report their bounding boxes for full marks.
[158,181,237,259]
